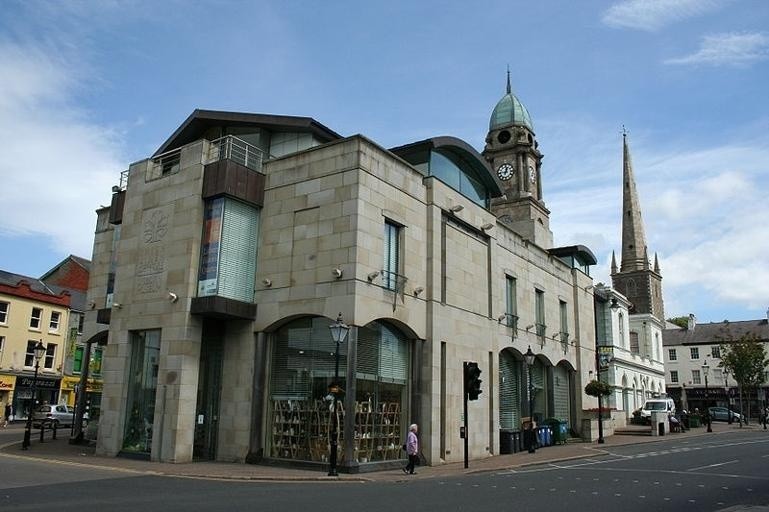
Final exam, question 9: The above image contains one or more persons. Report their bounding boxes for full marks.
[0,398,4,418]
[758,406,769,426]
[403,423,419,476]
[4,402,12,428]
[668,407,712,432]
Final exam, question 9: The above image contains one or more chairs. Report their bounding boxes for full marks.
[25,418,59,443]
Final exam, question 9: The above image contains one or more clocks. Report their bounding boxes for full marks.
[496,163,515,181]
[529,165,536,184]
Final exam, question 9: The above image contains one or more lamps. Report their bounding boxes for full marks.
[584,285,599,294]
[481,223,493,230]
[111,302,123,311]
[499,314,505,322]
[451,205,464,211]
[368,271,379,281]
[414,286,423,296]
[260,279,272,288]
[553,333,559,338]
[571,339,579,344]
[608,296,623,311]
[526,323,534,330]
[331,267,342,277]
[164,292,177,302]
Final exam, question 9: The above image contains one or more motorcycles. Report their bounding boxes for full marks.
[757,407,769,424]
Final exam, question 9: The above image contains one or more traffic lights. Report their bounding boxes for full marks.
[465,362,479,391]
[469,369,483,401]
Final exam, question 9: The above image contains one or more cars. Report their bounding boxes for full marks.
[707,406,742,423]
[33,405,89,428]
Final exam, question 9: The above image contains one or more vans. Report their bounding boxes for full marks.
[640,396,676,422]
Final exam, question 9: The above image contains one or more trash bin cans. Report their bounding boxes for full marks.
[680,414,702,428]
[500,416,568,455]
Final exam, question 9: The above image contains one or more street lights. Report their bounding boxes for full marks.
[17,338,47,451]
[325,311,350,476]
[590,288,622,445]
[721,367,732,424]
[524,344,538,453]
[702,359,714,434]
